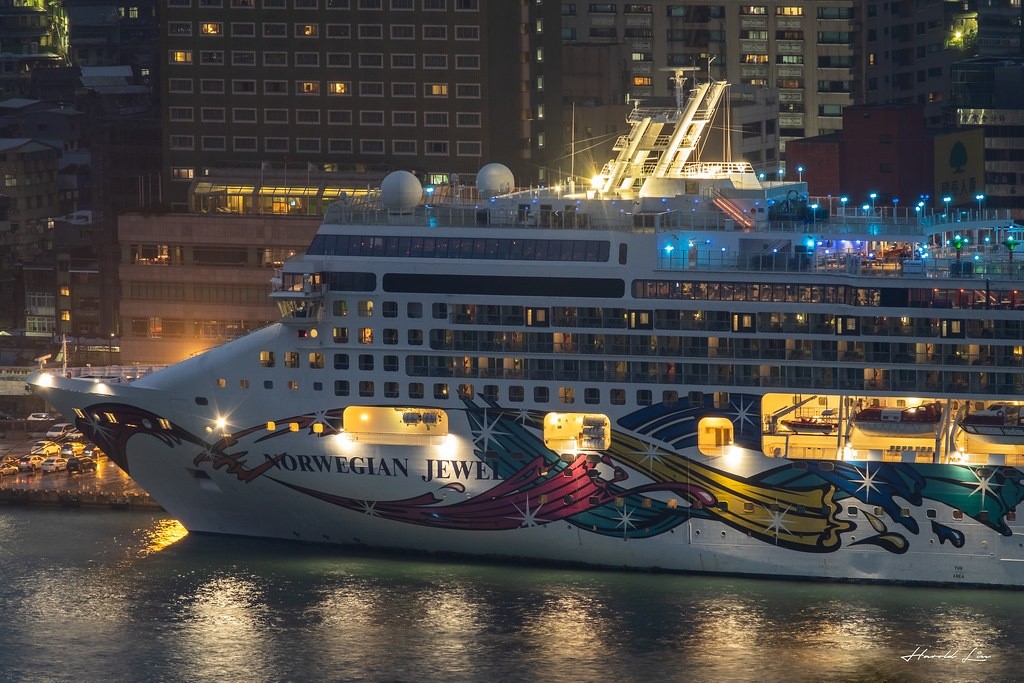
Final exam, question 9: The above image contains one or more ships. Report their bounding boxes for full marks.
[23,76,1024,591]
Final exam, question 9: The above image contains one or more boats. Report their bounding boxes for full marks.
[957,403,1024,438]
[849,399,946,437]
[779,415,840,435]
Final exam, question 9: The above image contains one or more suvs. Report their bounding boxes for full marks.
[67,455,97,475]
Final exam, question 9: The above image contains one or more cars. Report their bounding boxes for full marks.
[41,456,68,473]
[83,444,106,458]
[64,428,83,443]
[0,454,21,469]
[0,462,20,478]
[60,442,87,458]
[46,423,75,442]
[19,454,48,473]
[31,440,62,457]
[27,412,56,421]
[0,412,18,422]
